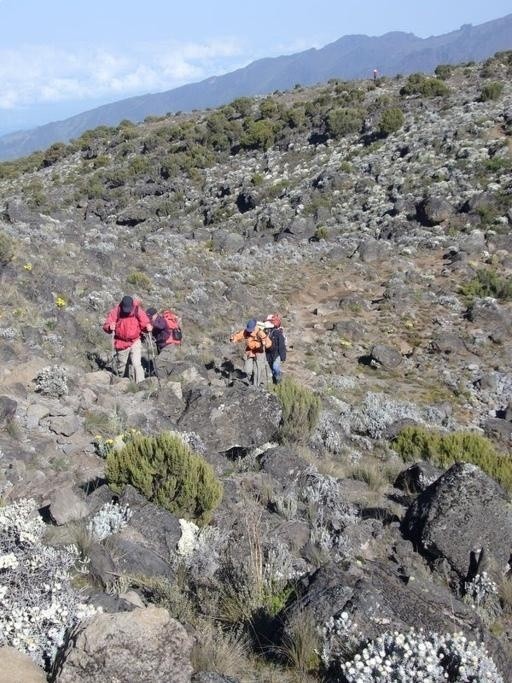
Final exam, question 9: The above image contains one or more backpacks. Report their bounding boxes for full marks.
[155,309,182,345]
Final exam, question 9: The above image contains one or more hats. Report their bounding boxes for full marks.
[247,321,256,333]
[121,296,134,314]
[146,307,158,319]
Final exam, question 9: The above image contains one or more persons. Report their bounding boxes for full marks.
[145,307,182,380]
[101,295,153,384]
[230,318,273,386]
[264,317,287,385]
